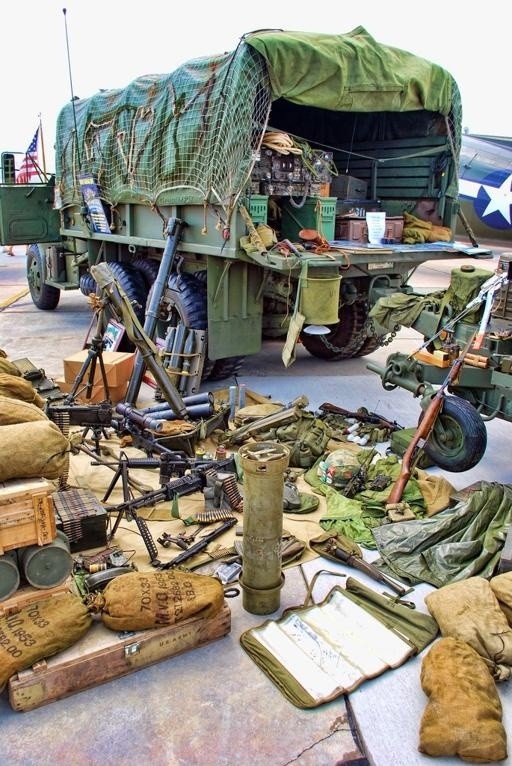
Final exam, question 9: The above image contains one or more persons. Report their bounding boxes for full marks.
[7,244,30,257]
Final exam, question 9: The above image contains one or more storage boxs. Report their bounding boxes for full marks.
[9,601,231,712]
[1,476,109,557]
[244,151,404,243]
[52,349,136,405]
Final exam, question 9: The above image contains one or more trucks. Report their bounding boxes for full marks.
[1,40,462,358]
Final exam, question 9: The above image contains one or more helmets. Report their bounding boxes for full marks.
[324,449,361,488]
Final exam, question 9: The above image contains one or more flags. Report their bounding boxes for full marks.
[14,124,44,184]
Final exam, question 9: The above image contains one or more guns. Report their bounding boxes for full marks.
[105,454,238,558]
[111,403,182,460]
[388,328,478,502]
[161,518,237,572]
[91,451,220,504]
[318,403,405,431]
[43,396,112,458]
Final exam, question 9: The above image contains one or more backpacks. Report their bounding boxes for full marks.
[236,402,330,467]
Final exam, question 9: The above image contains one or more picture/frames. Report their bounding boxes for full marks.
[142,338,166,389]
[103,319,125,351]
